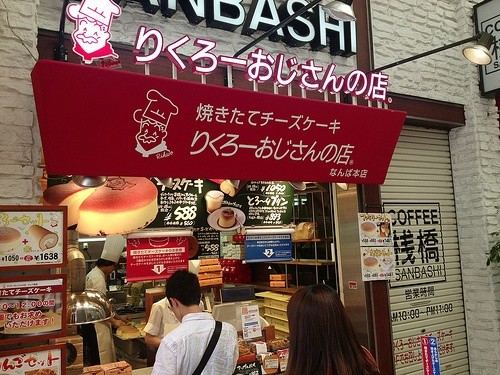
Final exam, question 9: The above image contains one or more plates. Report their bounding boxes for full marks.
[206,207,246,231]
[0,327,62,334]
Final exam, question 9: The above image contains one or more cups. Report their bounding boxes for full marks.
[205,190,224,214]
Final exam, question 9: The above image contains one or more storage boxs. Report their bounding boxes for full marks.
[270,274,291,287]
[197,259,253,287]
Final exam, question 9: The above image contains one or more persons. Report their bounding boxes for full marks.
[150,271,239,375]
[81,235,129,366]
[144,296,205,347]
[285,284,381,375]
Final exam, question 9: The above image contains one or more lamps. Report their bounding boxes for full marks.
[337,183,349,191]
[48,175,108,188]
[366,32,494,75]
[233,0,356,58]
[289,182,306,191]
[154,177,172,189]
[227,180,240,188]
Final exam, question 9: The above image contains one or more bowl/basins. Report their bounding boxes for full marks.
[361,222,394,272]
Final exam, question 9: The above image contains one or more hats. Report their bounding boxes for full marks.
[100,235,125,263]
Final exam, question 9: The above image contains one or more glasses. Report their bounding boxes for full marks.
[113,264,117,269]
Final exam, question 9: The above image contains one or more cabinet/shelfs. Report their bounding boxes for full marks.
[237,239,335,294]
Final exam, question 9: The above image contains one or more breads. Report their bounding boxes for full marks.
[0,227,21,252]
[41,175,158,237]
[270,339,289,348]
[239,342,250,355]
[116,319,147,339]
[292,222,318,240]
